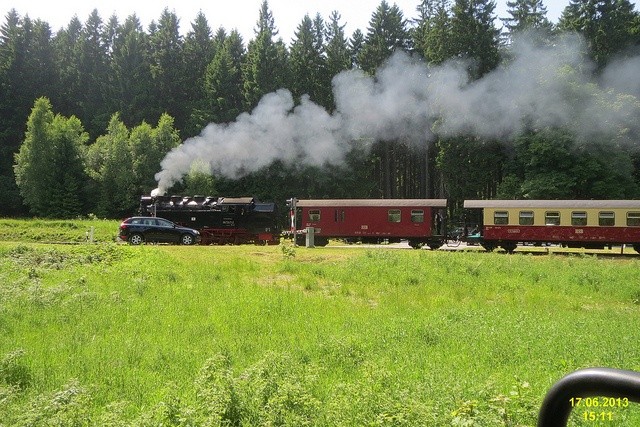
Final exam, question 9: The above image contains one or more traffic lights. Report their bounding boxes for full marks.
[285,198,293,209]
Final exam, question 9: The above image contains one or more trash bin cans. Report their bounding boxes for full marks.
[306,227,315,247]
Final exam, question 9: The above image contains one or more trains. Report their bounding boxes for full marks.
[137,195,640,252]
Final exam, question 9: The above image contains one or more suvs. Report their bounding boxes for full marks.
[119,216,200,245]
